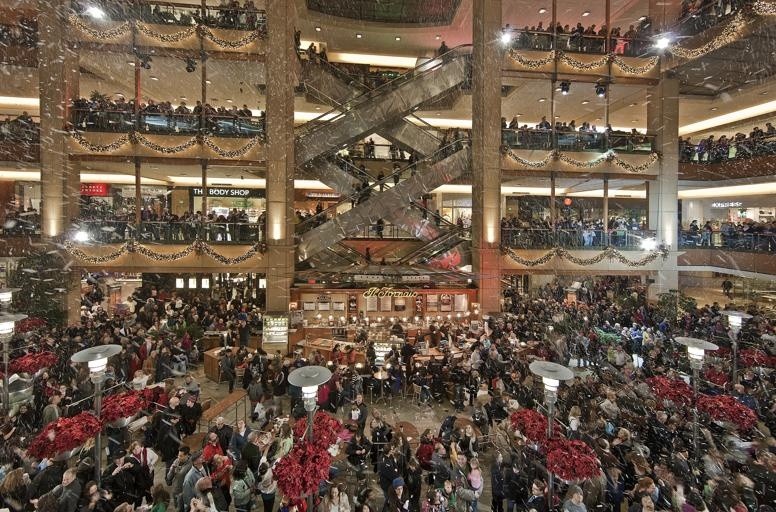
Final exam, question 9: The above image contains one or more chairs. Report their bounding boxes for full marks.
[412,383,424,406]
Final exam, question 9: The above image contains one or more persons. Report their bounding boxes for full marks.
[1,1,776,512]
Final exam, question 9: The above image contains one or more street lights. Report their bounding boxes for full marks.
[0,287,28,413]
[673,337,720,453]
[69,344,124,481]
[287,365,339,512]
[718,310,753,385]
[528,361,575,512]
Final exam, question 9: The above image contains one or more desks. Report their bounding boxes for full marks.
[200,388,249,434]
[375,372,390,405]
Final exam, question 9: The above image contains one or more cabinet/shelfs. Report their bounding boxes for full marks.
[203,346,240,385]
